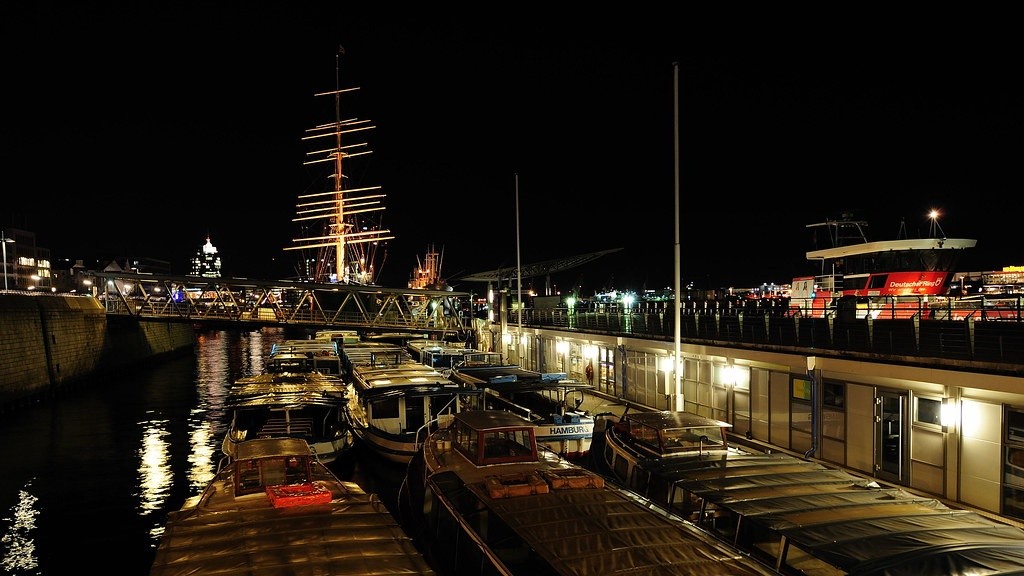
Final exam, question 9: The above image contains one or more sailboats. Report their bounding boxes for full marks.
[283,50,395,313]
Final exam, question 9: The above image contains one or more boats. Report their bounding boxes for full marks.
[155,437,433,576]
[407,340,465,379]
[402,246,445,305]
[412,390,781,576]
[605,402,1024,576]
[223,339,464,464]
[450,353,598,457]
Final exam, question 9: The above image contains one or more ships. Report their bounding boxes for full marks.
[743,209,977,319]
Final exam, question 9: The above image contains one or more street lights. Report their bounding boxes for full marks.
[2,238,15,291]
[931,211,938,237]
